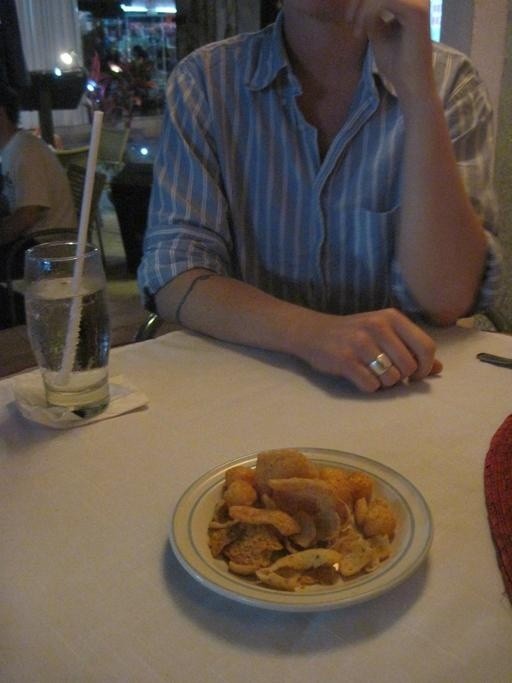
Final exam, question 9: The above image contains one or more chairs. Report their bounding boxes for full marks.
[96,127,130,172]
[0,164,107,324]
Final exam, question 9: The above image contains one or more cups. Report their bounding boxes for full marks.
[20,238,112,420]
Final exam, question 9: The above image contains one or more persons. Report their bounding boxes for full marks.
[1,84,78,283]
[108,21,169,71]
[137,0,504,394]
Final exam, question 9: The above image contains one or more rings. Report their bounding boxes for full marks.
[368,353,392,376]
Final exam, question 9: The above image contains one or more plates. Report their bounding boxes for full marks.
[166,445,439,615]
